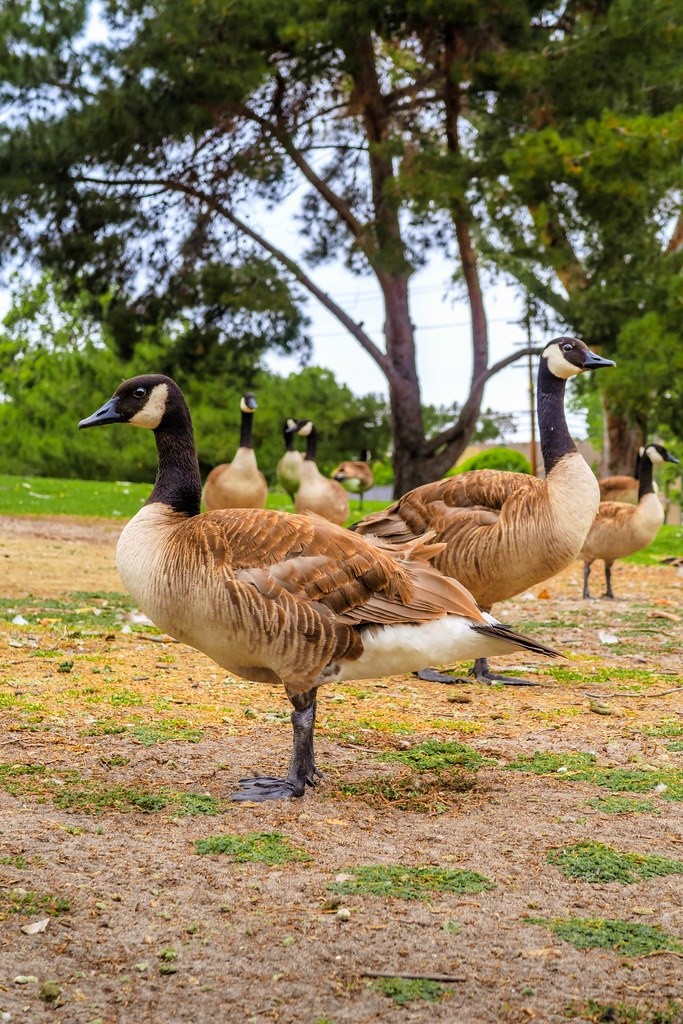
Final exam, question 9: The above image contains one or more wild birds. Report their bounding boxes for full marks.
[202,393,268,511]
[276,418,349,530]
[347,337,617,685]
[578,444,680,600]
[78,375,568,801]
[331,449,374,510]
